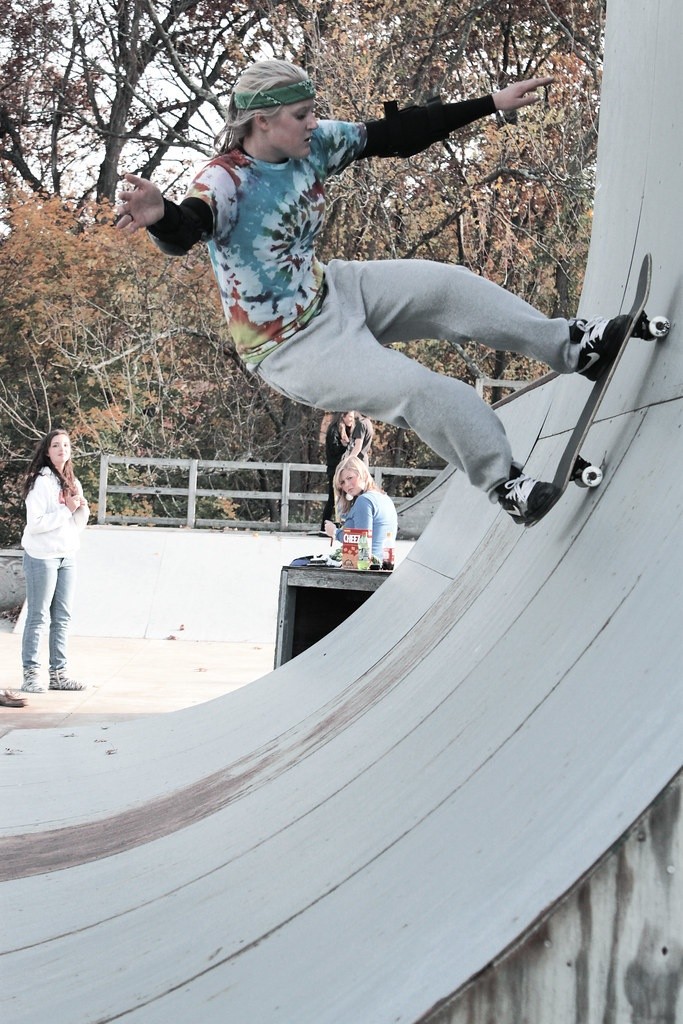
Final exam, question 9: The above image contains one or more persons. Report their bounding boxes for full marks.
[318,410,398,565]
[20,429,90,692]
[116,58,634,527]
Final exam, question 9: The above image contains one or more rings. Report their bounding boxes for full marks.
[128,213,135,222]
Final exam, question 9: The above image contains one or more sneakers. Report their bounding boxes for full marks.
[491,466,559,525]
[564,314,633,381]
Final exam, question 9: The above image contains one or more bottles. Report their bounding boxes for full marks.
[358,532,371,570]
[381,532,396,570]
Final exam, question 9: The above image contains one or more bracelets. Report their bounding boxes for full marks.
[333,529,337,540]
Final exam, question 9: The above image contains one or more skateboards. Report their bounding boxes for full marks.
[523,254,672,529]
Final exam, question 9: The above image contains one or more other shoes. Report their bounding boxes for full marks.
[48,668,84,691]
[319,531,330,537]
[0,690,28,707]
[22,667,44,692]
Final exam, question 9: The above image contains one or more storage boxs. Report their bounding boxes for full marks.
[342,528,372,569]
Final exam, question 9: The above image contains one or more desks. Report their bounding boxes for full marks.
[274,565,393,670]
